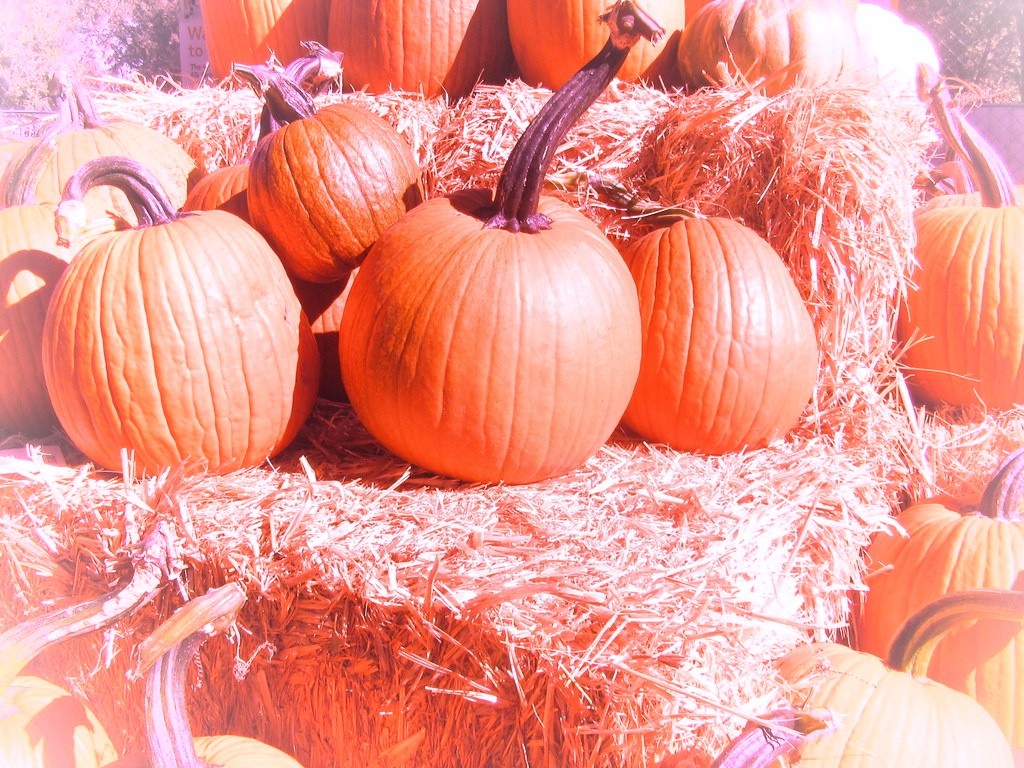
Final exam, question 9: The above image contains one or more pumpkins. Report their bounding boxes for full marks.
[694,445,1024,768]
[891,63,1024,413]
[201,0,844,98]
[0,41,818,484]
[0,522,311,768]
